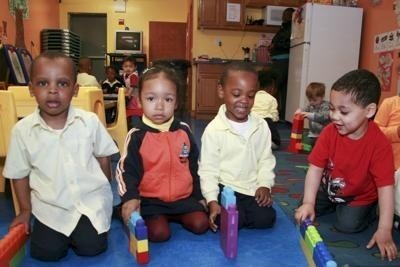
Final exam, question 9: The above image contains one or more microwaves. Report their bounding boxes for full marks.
[261,6,297,26]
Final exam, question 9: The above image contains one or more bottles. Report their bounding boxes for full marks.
[251,43,256,62]
[301,117,312,152]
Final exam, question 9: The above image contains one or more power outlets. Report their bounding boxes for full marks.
[216,39,222,46]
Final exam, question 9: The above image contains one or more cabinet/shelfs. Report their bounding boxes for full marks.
[198,0,245,31]
[104,52,146,87]
[195,62,229,120]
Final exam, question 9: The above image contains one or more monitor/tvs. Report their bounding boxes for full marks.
[114,30,142,54]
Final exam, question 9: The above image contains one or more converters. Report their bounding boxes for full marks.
[219,42,221,46]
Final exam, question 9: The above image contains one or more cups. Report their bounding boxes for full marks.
[242,46,250,62]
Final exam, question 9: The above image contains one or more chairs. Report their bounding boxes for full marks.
[0,89,20,217]
[107,88,128,156]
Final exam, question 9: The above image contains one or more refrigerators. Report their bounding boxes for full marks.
[285,3,364,124]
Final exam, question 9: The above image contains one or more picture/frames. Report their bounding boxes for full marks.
[17,46,35,84]
[2,44,29,85]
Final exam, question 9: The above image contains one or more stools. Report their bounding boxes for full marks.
[126,107,143,120]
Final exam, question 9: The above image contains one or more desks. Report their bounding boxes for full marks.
[103,92,128,109]
[8,86,105,130]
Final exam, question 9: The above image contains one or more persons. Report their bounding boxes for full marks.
[75,57,142,132]
[294,82,333,134]
[250,77,281,150]
[2,49,119,263]
[266,7,296,123]
[196,60,277,233]
[293,69,398,262]
[372,75,400,230]
[114,64,210,244]
